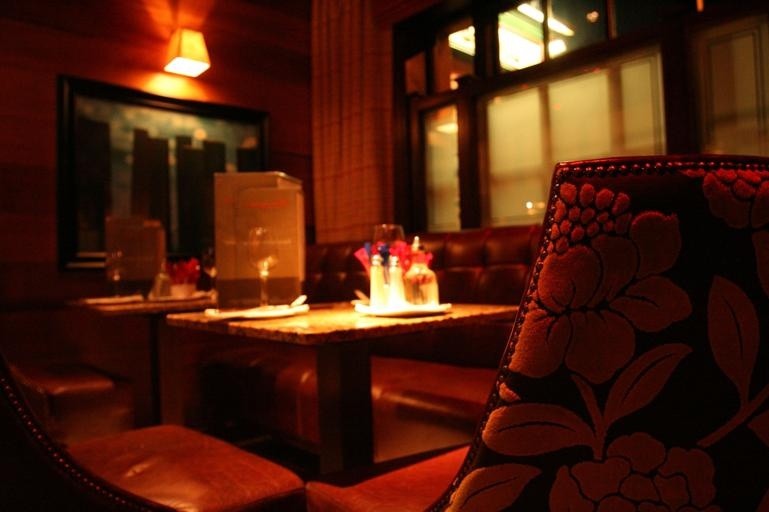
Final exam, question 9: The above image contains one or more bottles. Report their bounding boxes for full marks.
[370,254,386,307]
[404,256,441,306]
[389,256,406,304]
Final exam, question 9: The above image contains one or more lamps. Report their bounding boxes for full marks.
[164,28,211,77]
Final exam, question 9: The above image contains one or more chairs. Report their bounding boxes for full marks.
[304,152,769,512]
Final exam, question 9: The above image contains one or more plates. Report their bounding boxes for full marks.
[352,299,452,316]
[205,302,310,320]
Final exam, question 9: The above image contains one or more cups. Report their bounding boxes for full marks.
[373,223,406,247]
